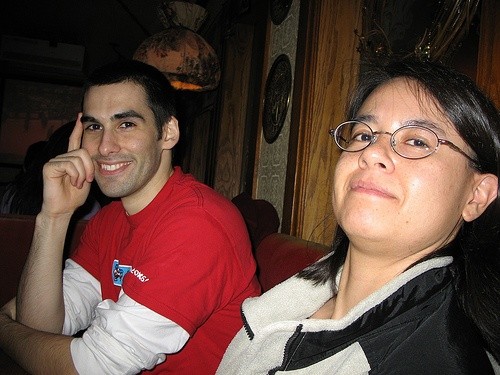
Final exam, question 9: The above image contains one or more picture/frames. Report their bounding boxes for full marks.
[353,0,480,69]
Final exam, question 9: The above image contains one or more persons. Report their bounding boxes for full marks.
[0,59,261,375]
[0,120,101,221]
[207,63,500,375]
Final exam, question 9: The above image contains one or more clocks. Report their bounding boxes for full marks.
[261,53,293,145]
[269,0,293,26]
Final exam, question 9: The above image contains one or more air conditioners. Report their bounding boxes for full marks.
[1,36,85,72]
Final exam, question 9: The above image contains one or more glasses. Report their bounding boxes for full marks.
[327,118,485,169]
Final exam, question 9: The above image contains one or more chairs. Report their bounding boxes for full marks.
[0,213,92,307]
[229,192,280,252]
[255,233,334,294]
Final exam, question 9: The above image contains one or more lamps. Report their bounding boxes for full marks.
[132,1,220,92]
